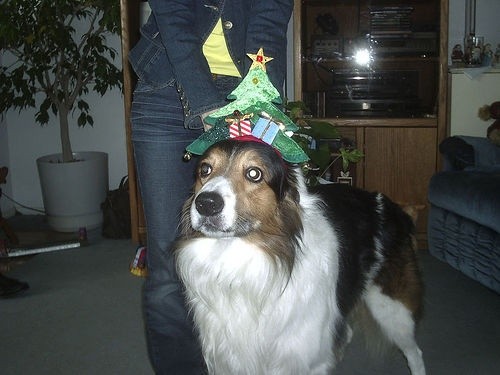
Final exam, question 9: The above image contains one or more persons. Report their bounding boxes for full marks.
[128,0,294,375]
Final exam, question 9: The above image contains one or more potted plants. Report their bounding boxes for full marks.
[0,0,123,232]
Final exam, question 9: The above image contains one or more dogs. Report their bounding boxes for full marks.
[161,140,427,374]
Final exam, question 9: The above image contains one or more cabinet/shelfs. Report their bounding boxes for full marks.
[120,0,449,249]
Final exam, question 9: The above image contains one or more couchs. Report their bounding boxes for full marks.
[428,136,500,292]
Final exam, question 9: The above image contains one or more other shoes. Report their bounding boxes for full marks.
[0,274,29,299]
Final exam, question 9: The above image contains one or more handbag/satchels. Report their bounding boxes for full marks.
[100,173,132,239]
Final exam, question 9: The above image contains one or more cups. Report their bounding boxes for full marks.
[464,36,484,68]
